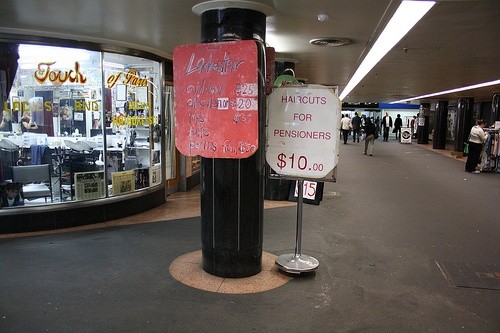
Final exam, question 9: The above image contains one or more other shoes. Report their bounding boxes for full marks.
[363,151,367,155]
[369,155,373,156]
[383,139,388,142]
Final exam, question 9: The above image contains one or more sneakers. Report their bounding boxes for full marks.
[357,140,359,143]
[344,141,347,144]
[353,140,355,142]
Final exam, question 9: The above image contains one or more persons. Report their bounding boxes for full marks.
[0,183,23,207]
[340,111,422,144]
[363,117,376,156]
[0,108,38,134]
[465,118,489,174]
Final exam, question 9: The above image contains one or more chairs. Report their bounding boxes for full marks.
[5,164,53,204]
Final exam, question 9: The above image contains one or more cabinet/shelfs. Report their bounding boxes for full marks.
[0,102,151,201]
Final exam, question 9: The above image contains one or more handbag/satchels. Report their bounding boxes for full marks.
[374,131,379,139]
[392,128,396,133]
